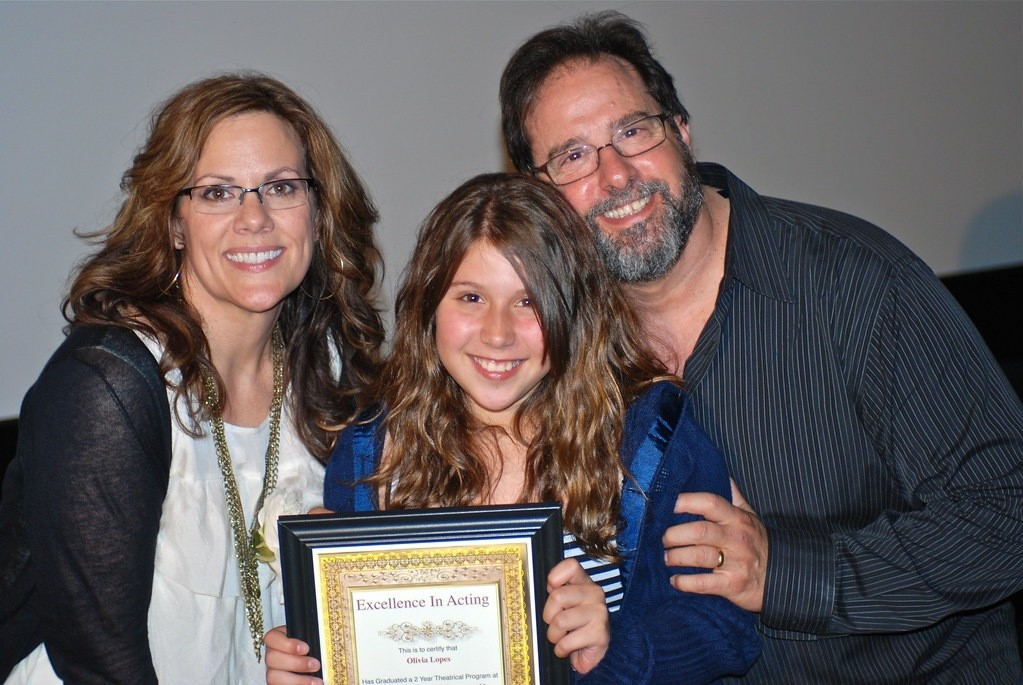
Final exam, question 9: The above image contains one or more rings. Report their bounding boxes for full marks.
[717,546,725,570]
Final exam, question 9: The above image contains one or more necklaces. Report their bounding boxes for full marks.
[186,318,295,667]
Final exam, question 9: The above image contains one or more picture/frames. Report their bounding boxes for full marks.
[277,501,571,685]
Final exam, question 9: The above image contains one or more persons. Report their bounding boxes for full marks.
[256,170,767,685]
[499,10,1021,683]
[2,68,384,685]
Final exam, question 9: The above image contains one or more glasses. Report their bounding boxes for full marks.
[527,110,672,186]
[177,178,316,215]
